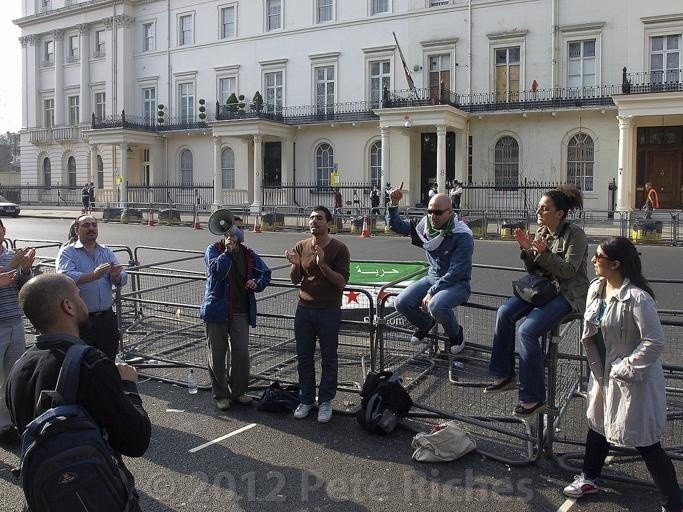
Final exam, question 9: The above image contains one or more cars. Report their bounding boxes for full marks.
[0,195,21,218]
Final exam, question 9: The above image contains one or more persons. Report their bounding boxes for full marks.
[0,217,37,435]
[483,185,589,418]
[284,205,351,424]
[54,214,128,363]
[384,181,475,355]
[5,272,151,512]
[81,183,96,211]
[332,179,463,214]
[562,235,683,512]
[641,182,660,219]
[199,215,273,412]
[0,268,17,289]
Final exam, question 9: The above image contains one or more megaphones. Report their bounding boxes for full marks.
[208,209,245,244]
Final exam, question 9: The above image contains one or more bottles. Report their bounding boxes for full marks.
[188,368,197,394]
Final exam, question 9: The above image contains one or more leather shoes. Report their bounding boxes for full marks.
[483,369,516,395]
[513,400,544,416]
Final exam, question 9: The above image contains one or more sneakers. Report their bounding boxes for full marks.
[0,428,18,445]
[410,318,435,346]
[292,401,312,419]
[214,397,229,410]
[237,393,253,404]
[316,399,332,424]
[448,325,465,354]
[561,471,601,498]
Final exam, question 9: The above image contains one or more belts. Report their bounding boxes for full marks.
[88,310,105,319]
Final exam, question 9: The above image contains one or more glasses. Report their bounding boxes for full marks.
[425,208,450,216]
[594,251,613,263]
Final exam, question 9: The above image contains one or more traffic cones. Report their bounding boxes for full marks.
[145,210,157,227]
[360,216,371,238]
[253,215,263,234]
[193,211,204,230]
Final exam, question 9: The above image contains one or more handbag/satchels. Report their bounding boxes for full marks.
[410,420,475,466]
[359,369,414,434]
[511,272,562,308]
[256,379,304,417]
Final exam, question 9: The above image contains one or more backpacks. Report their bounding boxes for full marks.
[13,340,137,512]
